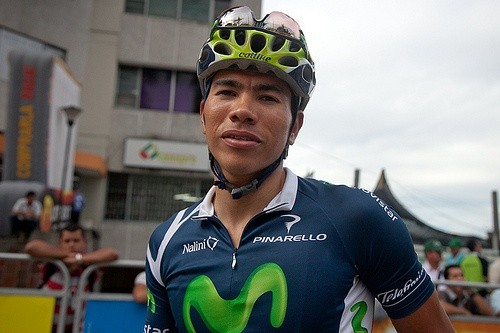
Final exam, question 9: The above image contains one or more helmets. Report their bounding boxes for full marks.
[197,5,317,102]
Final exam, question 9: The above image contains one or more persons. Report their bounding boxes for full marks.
[132,270,148,303]
[24,223,120,333]
[421,236,500,317]
[10,190,42,244]
[144,5,456,333]
[71,185,85,230]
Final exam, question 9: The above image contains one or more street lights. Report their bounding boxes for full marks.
[56,105,82,233]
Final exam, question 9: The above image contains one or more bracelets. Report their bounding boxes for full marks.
[75,252,83,266]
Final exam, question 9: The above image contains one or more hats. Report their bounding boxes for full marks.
[424,238,445,252]
[449,237,465,247]
